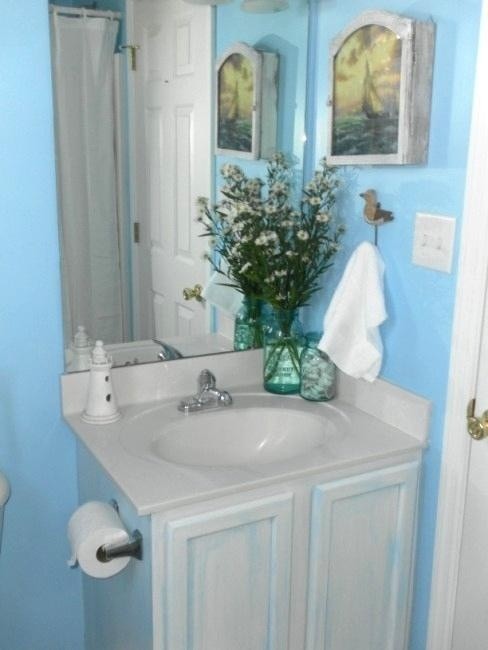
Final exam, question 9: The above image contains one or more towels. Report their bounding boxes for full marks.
[317,242,387,383]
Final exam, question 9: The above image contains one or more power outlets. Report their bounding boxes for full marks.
[411,212,455,273]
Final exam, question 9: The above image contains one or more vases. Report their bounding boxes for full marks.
[263,303,305,395]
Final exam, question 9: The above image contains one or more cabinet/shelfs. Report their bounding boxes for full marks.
[75,458,418,650]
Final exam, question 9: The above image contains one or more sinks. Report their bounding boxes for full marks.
[122,394,352,476]
[107,342,231,366]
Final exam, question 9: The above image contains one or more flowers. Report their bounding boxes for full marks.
[221,161,345,390]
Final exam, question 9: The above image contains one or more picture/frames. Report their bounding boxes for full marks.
[328,9,436,166]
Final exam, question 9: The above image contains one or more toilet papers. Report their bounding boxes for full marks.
[67,501,131,578]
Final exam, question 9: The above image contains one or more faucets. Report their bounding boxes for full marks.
[177,367,234,412]
[150,337,183,362]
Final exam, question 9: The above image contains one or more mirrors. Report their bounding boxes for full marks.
[46,0,313,376]
